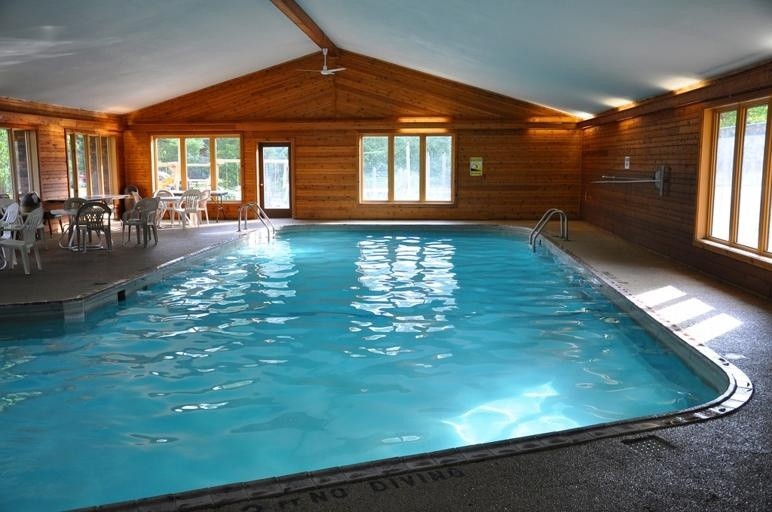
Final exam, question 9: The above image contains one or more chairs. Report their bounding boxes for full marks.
[0,186,231,276]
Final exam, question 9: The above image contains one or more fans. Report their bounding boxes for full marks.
[294,46,348,78]
